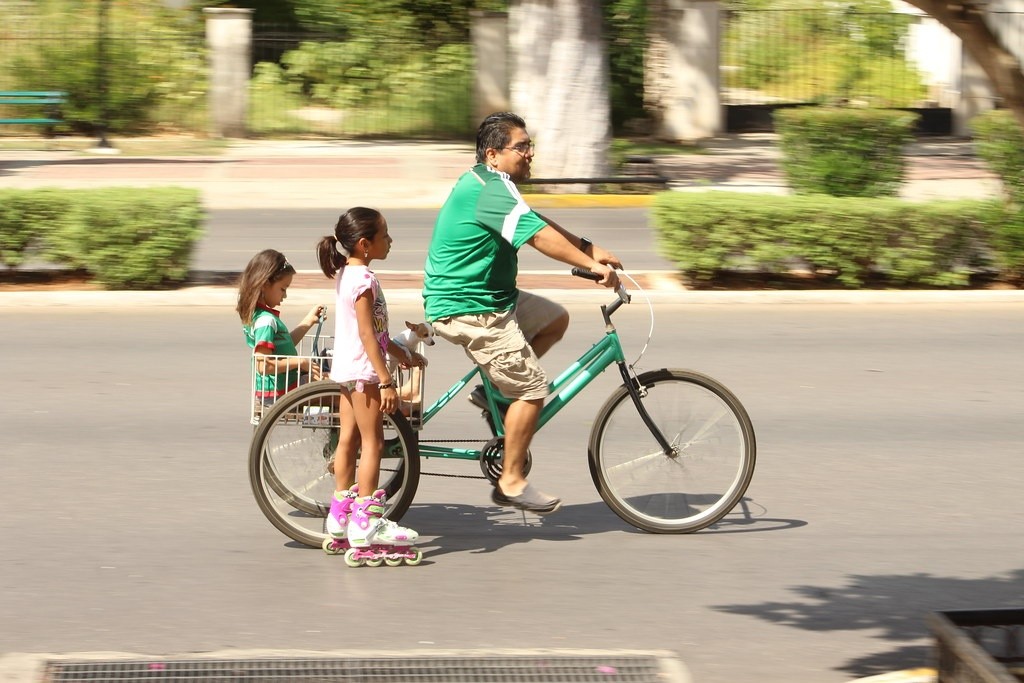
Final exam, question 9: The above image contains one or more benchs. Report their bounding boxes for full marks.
[0,91,70,139]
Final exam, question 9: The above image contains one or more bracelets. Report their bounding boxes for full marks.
[378,380,396,389]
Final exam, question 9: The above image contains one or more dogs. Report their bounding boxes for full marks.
[385,321,435,375]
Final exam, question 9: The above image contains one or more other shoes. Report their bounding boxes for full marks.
[492,481,560,511]
[468,383,509,421]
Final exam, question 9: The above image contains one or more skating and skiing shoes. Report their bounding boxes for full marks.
[322,482,359,554]
[345,489,423,567]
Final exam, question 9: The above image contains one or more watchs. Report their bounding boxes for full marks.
[580,236,592,252]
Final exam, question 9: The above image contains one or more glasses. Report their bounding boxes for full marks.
[492,143,535,154]
[268,260,291,281]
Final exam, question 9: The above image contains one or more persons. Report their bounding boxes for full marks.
[235,250,423,416]
[422,110,624,515]
[317,207,419,547]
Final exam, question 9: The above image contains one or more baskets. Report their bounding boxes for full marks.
[251,325,425,431]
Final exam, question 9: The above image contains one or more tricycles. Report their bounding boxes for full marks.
[248,264,757,551]
